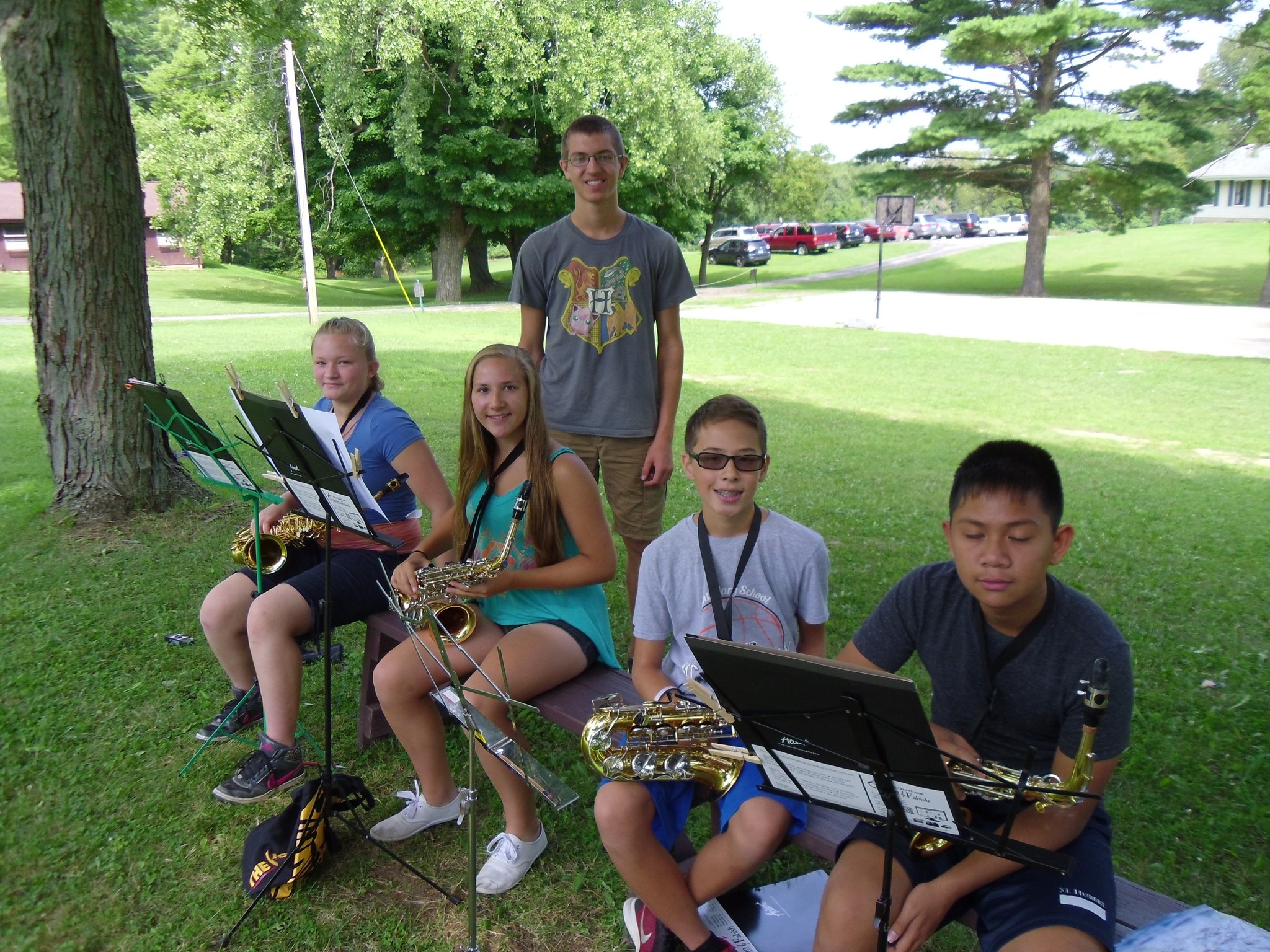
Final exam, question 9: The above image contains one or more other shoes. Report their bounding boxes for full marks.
[369,788,470,843]
[476,817,548,894]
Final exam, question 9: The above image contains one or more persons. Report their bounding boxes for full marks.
[193,316,459,803]
[366,341,618,896]
[504,112,697,676]
[811,440,1134,952]
[592,393,831,952]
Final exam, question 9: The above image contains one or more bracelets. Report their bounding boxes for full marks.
[653,686,682,702]
[409,550,428,561]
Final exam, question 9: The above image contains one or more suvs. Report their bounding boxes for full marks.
[829,222,865,250]
[908,213,939,241]
[698,225,760,251]
[708,239,771,267]
[761,222,837,255]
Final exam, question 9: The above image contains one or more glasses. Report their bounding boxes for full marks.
[688,451,767,472]
[564,152,623,167]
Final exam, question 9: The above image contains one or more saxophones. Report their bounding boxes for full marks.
[388,479,534,645]
[849,654,1110,857]
[232,471,410,573]
[581,686,743,802]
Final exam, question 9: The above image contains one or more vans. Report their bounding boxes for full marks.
[938,213,981,237]
[995,214,1029,235]
[755,223,781,237]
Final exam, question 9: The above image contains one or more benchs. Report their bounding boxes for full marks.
[357,613,1268,952]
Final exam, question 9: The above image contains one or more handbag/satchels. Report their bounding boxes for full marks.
[243,773,376,900]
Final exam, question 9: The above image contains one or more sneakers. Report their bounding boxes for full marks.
[623,896,677,952]
[212,731,307,805]
[195,676,264,741]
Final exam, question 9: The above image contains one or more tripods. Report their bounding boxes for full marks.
[214,513,464,951]
[169,494,341,786]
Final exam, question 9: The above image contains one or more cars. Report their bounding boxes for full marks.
[937,218,960,240]
[981,217,1016,238]
[857,220,896,243]
